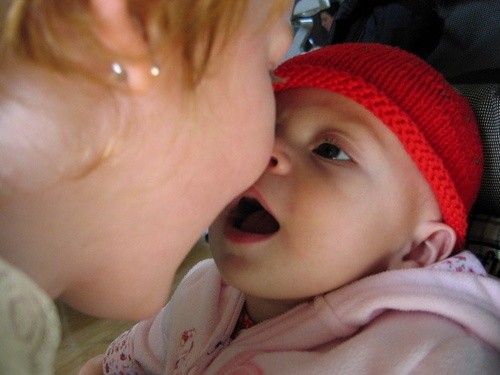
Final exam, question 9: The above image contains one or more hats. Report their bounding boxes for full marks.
[272,42,484,254]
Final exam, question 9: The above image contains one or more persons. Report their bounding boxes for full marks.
[0,1,295,375]
[79,44,500,375]
[302,0,500,84]
